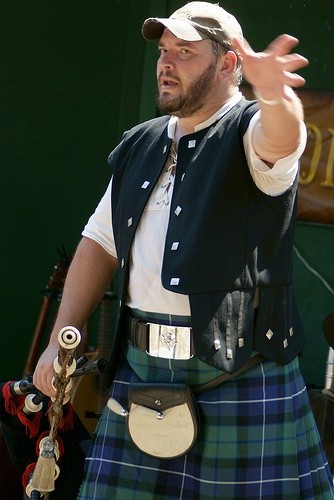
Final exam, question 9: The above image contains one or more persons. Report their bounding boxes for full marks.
[32,2,334,500]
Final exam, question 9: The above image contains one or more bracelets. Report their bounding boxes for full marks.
[252,86,284,105]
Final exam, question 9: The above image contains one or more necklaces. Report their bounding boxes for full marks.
[161,139,178,205]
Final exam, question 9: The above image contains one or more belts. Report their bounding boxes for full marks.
[125,316,197,361]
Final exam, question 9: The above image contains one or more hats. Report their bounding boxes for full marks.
[142,2,243,58]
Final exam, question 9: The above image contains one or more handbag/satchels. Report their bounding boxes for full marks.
[127,384,201,461]
